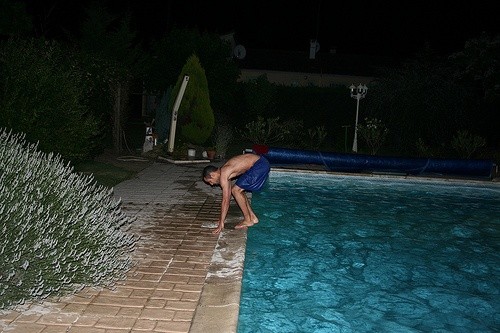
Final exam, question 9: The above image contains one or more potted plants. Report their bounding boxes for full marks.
[187,146,215,158]
[215,126,231,160]
[244,116,290,154]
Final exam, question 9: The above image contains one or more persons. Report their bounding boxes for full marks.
[202,153,270,235]
[490,163,498,182]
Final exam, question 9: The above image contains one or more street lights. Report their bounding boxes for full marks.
[349,82,368,152]
